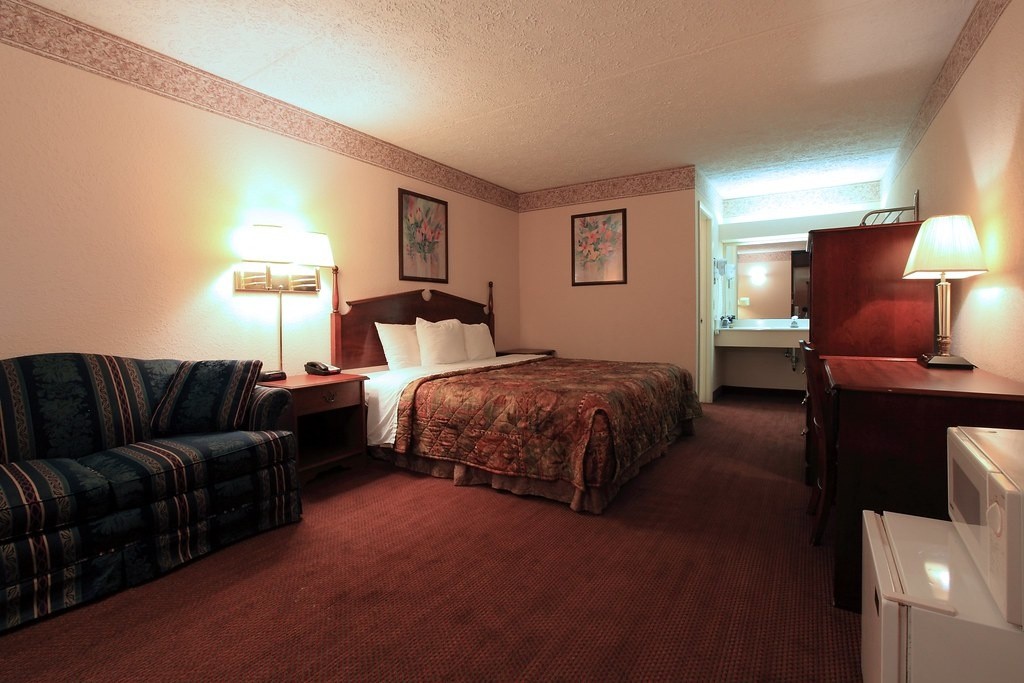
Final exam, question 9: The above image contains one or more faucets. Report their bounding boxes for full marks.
[789,316,800,327]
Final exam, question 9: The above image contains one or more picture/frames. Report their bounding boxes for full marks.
[398,188,448,283]
[570,208,627,285]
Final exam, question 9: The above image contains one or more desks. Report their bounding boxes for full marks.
[815,356,1024,611]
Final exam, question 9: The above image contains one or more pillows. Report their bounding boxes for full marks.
[463,323,496,361]
[416,317,469,367]
[374,322,421,370]
[150,359,263,436]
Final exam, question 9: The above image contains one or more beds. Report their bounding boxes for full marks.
[341,354,704,514]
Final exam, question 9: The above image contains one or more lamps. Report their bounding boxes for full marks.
[242,223,293,291]
[292,231,335,292]
[901,214,989,370]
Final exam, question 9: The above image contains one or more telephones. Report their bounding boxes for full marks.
[304,361,342,376]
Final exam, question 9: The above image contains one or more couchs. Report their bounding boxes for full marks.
[0,352,304,634]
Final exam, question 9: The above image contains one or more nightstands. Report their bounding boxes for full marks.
[497,348,556,356]
[255,372,371,479]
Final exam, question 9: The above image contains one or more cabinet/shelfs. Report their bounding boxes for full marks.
[857,508,1024,683]
[790,249,811,320]
[799,220,936,490]
